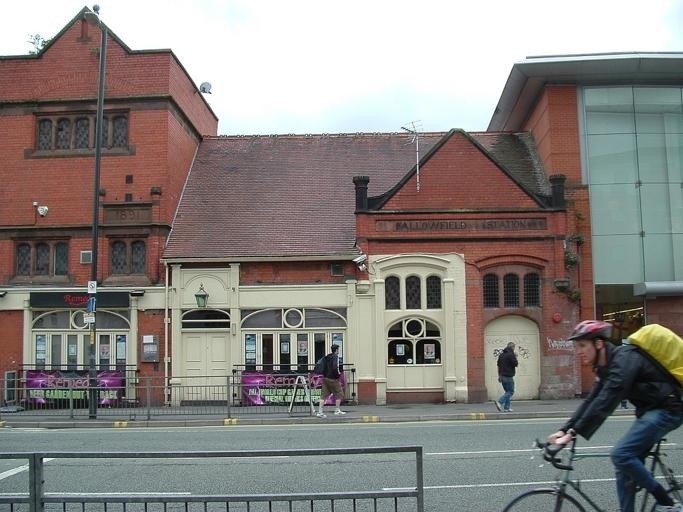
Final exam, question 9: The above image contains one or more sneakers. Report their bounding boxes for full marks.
[333,409,346,415]
[316,412,328,418]
[656,499,682,511]
[504,408,514,411]
[495,400,502,411]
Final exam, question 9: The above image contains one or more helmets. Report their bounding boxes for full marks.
[567,318,612,339]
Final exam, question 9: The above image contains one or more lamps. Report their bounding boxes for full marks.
[193,283,208,308]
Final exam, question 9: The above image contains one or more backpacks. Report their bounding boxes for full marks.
[315,356,326,374]
[616,323,683,396]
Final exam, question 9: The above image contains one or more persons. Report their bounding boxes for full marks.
[316,344,346,418]
[494,341,519,412]
[547,319,683,511]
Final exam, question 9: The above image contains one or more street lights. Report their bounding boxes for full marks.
[83,4,108,419]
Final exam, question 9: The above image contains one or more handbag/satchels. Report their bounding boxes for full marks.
[498,374,501,382]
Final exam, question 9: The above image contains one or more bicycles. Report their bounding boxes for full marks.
[502,432,683,512]
